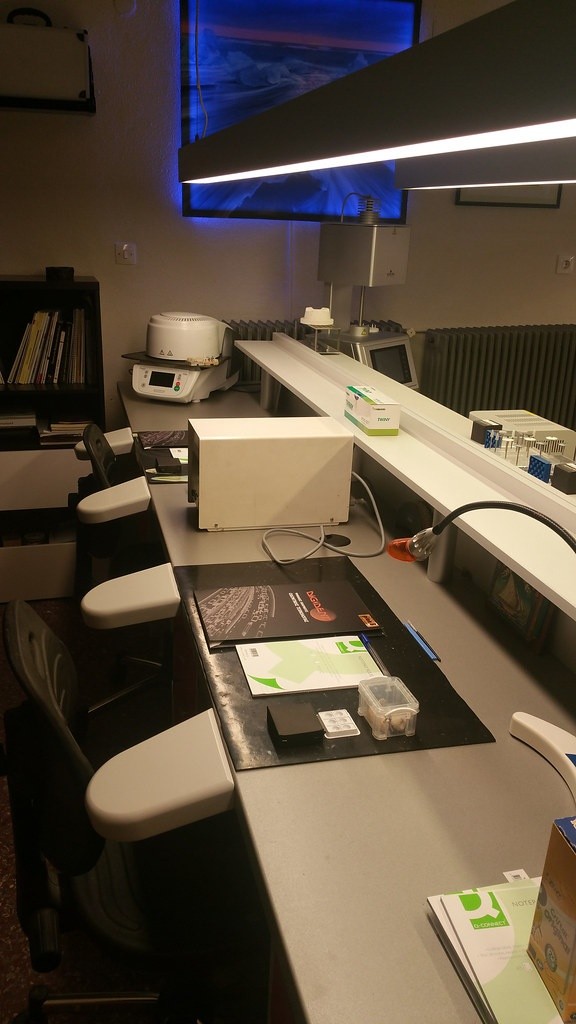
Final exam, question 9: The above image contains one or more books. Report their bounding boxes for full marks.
[235,635,384,698]
[7,306,86,384]
[424,872,566,1024]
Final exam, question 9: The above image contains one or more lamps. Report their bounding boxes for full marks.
[178,0,576,184]
[394,137,576,190]
[386,500,576,564]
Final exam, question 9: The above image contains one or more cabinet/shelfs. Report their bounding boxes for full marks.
[0,275,112,594]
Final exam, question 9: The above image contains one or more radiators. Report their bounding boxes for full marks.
[420,324,576,431]
[222,318,406,382]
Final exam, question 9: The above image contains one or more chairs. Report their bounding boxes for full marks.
[82,423,137,489]
[5,597,173,782]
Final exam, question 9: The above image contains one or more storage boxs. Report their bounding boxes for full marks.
[0,510,79,604]
[267,702,326,749]
[344,384,402,436]
[526,816,576,1024]
[355,676,421,739]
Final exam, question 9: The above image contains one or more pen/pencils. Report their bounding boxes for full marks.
[358,632,391,677]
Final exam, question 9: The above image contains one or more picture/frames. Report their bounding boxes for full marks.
[489,560,560,656]
[455,185,562,209]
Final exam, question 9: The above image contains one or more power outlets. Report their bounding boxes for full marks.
[114,242,139,265]
[556,255,574,274]
